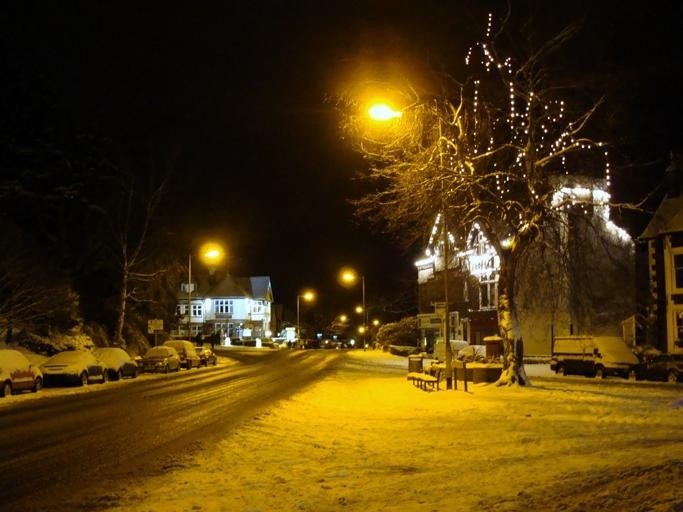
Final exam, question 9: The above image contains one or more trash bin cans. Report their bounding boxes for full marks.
[408,354,424,373]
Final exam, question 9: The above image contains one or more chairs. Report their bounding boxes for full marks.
[407,366,442,391]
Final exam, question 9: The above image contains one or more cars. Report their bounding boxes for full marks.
[38,349,108,386]
[318,335,358,349]
[93,338,217,382]
[628,352,683,381]
[0,349,43,398]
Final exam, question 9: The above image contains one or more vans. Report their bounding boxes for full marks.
[550,333,640,381]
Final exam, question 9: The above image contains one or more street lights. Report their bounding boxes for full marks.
[342,273,368,348]
[187,248,221,343]
[366,99,458,392]
[296,294,313,337]
